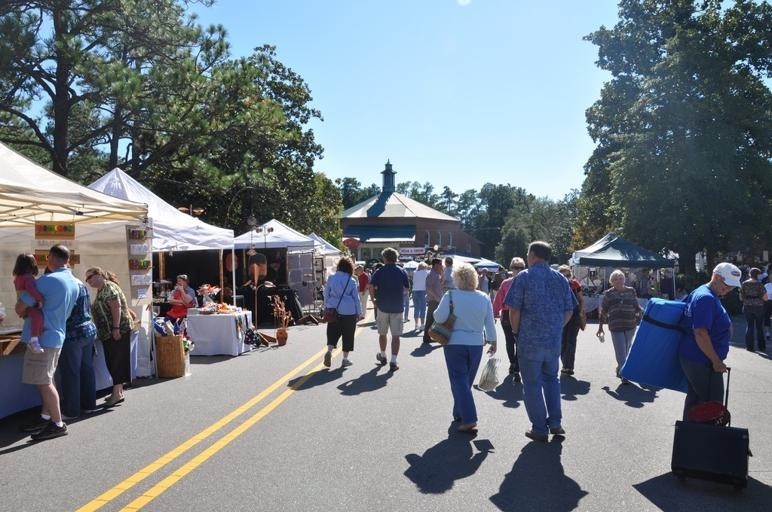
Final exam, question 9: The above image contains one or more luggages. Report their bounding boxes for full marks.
[671,367,754,488]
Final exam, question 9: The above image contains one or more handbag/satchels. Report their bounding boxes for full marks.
[322,274,353,324]
[427,288,458,347]
[575,307,587,332]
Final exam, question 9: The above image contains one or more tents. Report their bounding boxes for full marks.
[0,135,152,380]
[308,228,342,262]
[234,217,322,326]
[571,231,679,293]
[85,166,234,376]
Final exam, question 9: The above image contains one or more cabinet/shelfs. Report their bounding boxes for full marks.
[0,327,140,421]
[582,296,650,320]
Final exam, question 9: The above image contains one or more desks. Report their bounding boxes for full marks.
[187,311,252,356]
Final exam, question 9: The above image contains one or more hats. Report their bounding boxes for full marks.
[712,261,742,289]
[176,274,188,281]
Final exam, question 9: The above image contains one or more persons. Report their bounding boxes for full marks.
[441,257,455,291]
[509,242,578,442]
[743,269,768,347]
[422,258,443,344]
[322,257,362,371]
[45,266,97,418]
[494,259,525,385]
[557,266,584,373]
[679,262,742,427]
[596,269,644,385]
[18,246,79,440]
[356,258,509,319]
[412,260,429,332]
[434,264,497,437]
[86,268,133,408]
[168,275,196,331]
[14,254,45,351]
[372,248,412,371]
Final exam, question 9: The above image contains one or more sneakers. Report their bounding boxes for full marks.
[457,421,477,434]
[508,363,515,377]
[389,361,400,372]
[525,430,549,444]
[620,375,630,386]
[415,325,418,331]
[18,415,52,434]
[30,418,70,442]
[514,371,522,384]
[453,415,462,422]
[561,367,574,374]
[376,352,387,366]
[323,351,331,368]
[420,325,425,331]
[550,426,566,436]
[342,359,352,367]
[104,396,126,407]
[105,397,113,401]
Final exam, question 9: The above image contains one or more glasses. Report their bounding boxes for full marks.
[85,272,99,283]
[564,273,570,279]
[511,265,526,271]
[721,278,737,289]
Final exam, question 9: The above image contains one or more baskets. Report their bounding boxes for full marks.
[154,333,186,378]
